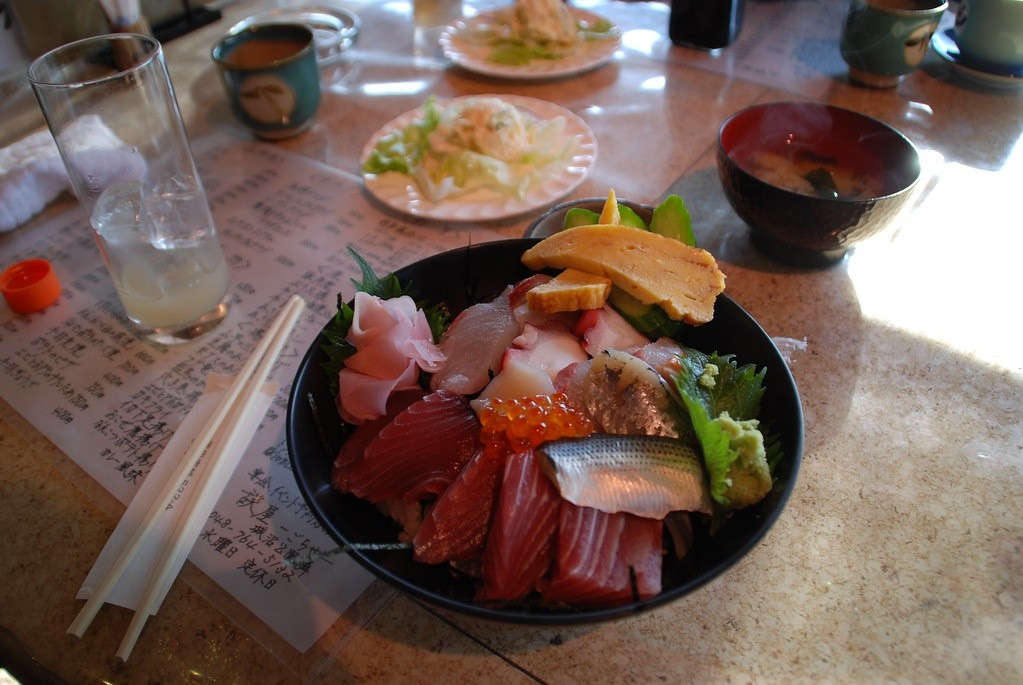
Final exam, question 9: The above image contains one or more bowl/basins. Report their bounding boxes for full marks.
[717,102,925,266]
[524,197,668,239]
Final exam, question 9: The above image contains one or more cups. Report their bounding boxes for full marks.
[952,0,1023,75]
[27,30,235,343]
[211,21,324,143]
[840,0,949,86]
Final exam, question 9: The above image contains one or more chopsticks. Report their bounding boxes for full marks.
[62,294,306,663]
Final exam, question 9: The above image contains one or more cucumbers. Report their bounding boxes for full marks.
[563,195,696,339]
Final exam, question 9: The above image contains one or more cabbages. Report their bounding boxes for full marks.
[451,10,620,66]
[358,93,575,200]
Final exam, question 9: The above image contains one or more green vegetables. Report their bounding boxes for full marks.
[669,346,770,506]
[316,242,449,398]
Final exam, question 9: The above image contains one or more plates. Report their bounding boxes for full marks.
[362,93,599,224]
[285,235,804,628]
[926,24,1023,91]
[222,6,360,63]
[441,4,622,78]
